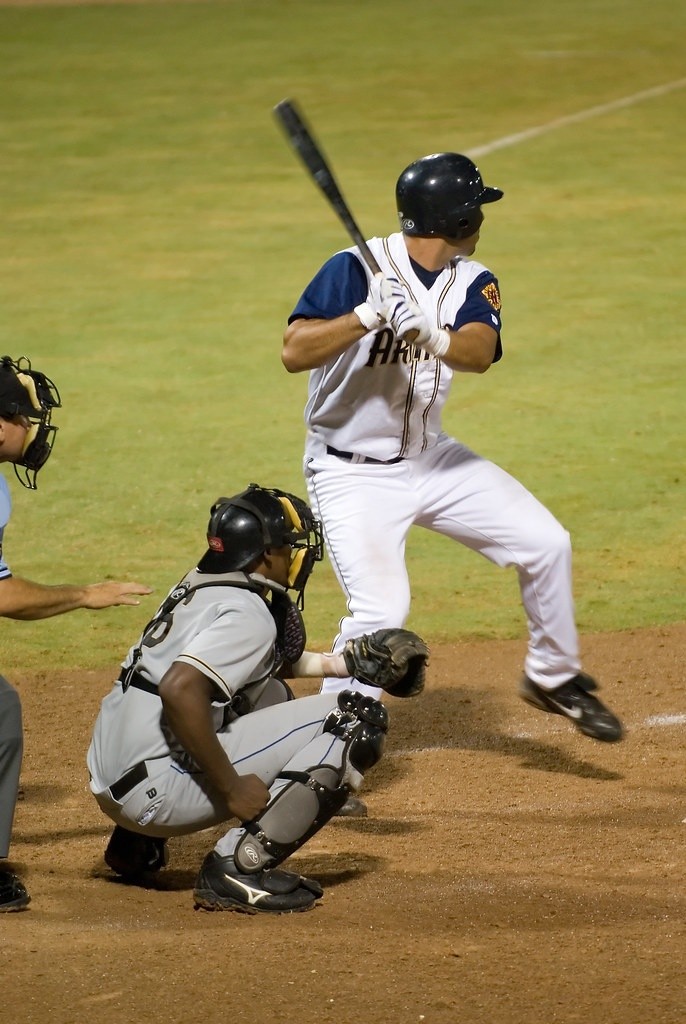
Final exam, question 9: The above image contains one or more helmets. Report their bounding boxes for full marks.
[196,480,293,576]
[396,152,504,239]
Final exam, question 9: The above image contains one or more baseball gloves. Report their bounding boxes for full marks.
[343,628,432,698]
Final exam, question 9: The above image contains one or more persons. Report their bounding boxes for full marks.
[282,151,622,815]
[88,482,427,913]
[0,355,153,912]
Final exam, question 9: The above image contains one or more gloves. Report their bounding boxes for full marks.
[385,299,450,359]
[352,272,406,331]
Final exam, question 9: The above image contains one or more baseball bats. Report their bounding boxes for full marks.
[271,98,419,343]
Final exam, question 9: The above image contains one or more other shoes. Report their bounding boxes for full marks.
[0,870,31,913]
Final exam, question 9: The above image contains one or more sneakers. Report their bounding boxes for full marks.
[192,851,325,915]
[105,829,171,875]
[521,672,623,742]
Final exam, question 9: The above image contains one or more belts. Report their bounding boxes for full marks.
[109,762,149,801]
[326,445,404,465]
